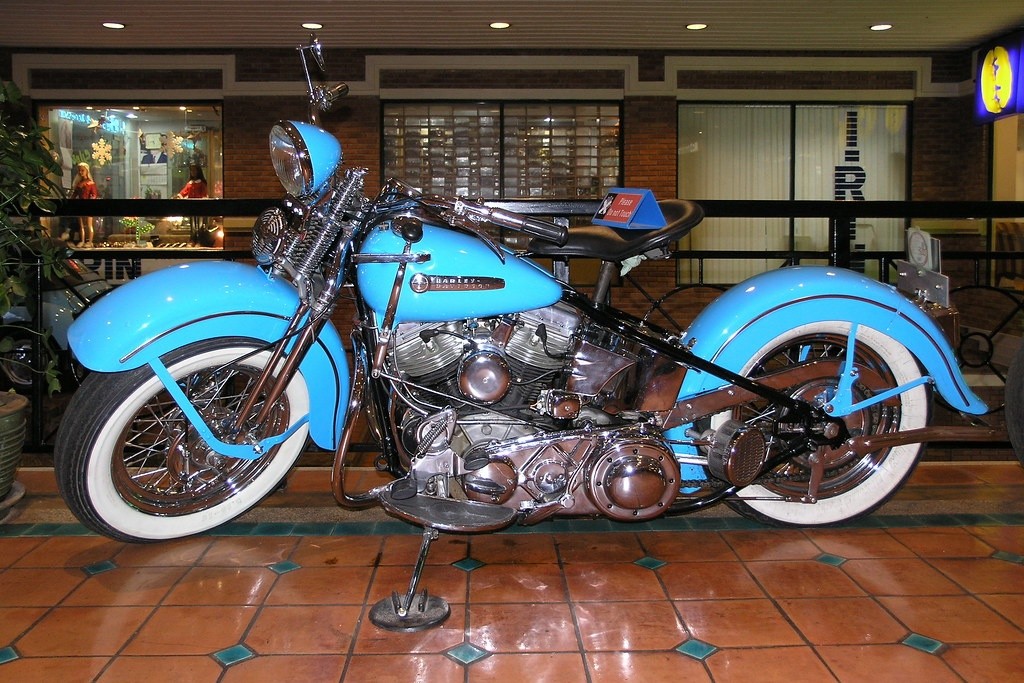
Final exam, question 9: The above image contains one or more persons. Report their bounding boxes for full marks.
[71,161,98,248]
[170,162,207,240]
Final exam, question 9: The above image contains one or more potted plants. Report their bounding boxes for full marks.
[0,81,79,526]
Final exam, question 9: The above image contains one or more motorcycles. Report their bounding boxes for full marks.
[50,32,990,637]
